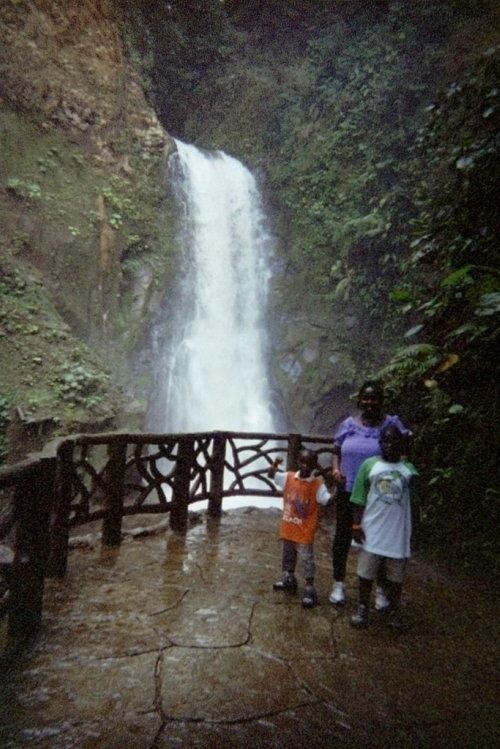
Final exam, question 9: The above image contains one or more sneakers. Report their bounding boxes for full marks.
[272,577,298,589]
[301,583,318,608]
[329,581,346,605]
[374,588,391,611]
[350,605,369,627]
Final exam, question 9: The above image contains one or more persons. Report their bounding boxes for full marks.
[330,379,395,611]
[345,417,425,630]
[267,446,343,608]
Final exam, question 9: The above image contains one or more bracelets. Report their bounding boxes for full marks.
[352,523,362,531]
